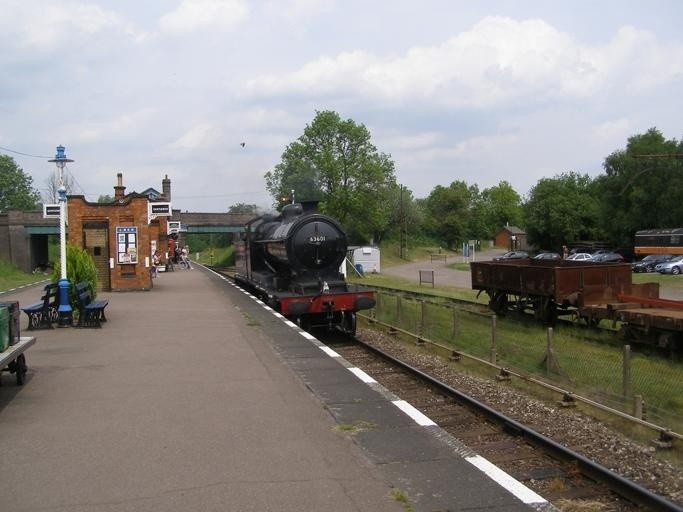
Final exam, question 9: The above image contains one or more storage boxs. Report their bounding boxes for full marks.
[0,307,9,351]
[0,301,19,344]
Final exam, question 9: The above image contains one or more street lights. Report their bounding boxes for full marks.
[47,144,76,328]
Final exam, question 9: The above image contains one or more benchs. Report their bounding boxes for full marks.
[21,284,59,329]
[75,282,109,328]
[178,261,188,270]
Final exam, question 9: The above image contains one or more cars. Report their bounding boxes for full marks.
[585,250,625,263]
[493,250,529,260]
[534,253,562,262]
[565,253,592,263]
[632,255,683,275]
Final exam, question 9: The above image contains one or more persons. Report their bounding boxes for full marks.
[151,245,196,279]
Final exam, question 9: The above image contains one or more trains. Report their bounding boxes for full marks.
[634,229,683,255]
[469,257,683,356]
[234,202,379,338]
